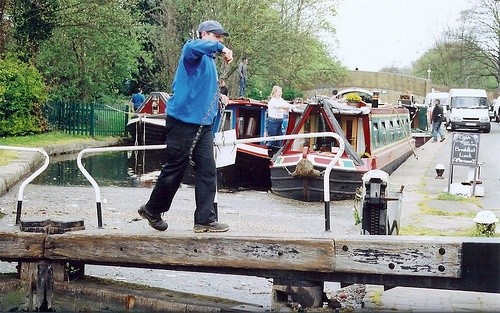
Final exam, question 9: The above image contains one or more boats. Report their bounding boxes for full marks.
[268,90,415,202]
[126,91,171,145]
[216,96,308,184]
[395,94,432,148]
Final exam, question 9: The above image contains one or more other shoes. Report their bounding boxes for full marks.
[440,138,445,142]
[432,141,437,143]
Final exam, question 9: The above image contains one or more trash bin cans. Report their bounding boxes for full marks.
[419,106,428,130]
[406,105,418,128]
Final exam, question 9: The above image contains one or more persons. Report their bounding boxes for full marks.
[237,58,248,99]
[131,88,145,111]
[430,99,446,143]
[220,80,229,97]
[266,86,296,147]
[138,20,233,233]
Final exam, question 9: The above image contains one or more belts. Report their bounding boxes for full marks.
[269,117,282,121]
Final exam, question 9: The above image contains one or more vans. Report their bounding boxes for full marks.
[424,92,451,128]
[447,88,491,133]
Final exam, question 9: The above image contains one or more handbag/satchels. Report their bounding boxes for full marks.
[211,129,237,168]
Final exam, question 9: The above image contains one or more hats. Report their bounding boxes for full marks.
[434,99,440,102]
[198,21,229,36]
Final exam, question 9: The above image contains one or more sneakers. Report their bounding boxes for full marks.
[194,222,229,233]
[138,206,168,231]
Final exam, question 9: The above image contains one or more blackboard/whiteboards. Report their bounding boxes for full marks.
[450,133,480,166]
